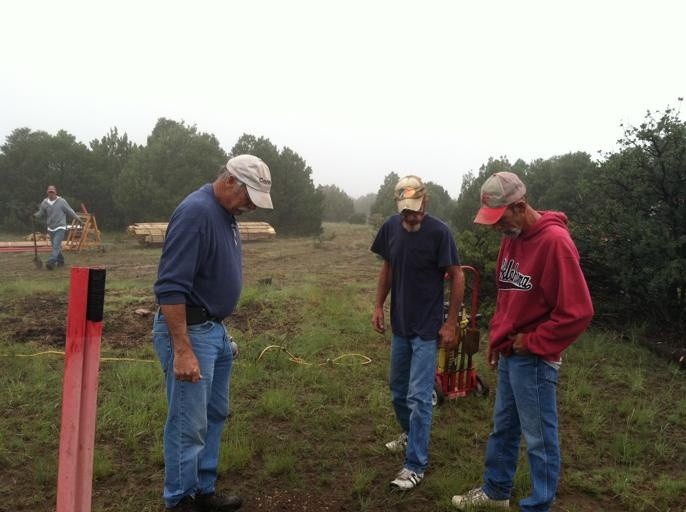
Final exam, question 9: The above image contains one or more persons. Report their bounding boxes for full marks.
[147,152,277,510]
[448,170,595,512]
[369,175,467,494]
[31,184,86,269]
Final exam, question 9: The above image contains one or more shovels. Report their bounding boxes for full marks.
[32,216,43,269]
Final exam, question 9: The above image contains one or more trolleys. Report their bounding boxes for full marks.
[431,264,489,406]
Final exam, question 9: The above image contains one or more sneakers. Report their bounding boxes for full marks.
[165,495,208,512]
[451,487,509,512]
[196,491,242,512]
[389,467,424,492]
[385,432,408,454]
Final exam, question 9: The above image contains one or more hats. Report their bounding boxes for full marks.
[47,186,56,192]
[474,172,526,225]
[394,176,426,213]
[226,154,274,209]
